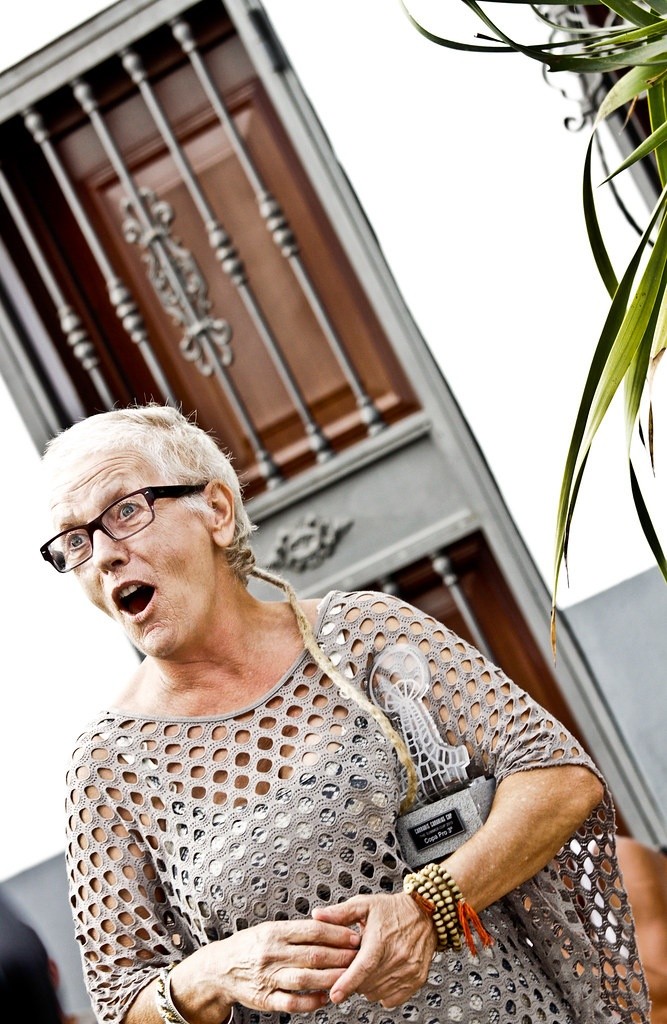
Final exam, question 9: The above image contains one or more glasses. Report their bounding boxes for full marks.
[40,483,208,573]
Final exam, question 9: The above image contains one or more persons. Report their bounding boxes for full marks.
[41,406,652,1024]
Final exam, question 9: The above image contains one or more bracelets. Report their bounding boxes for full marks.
[403,863,493,958]
[155,962,234,1024]
